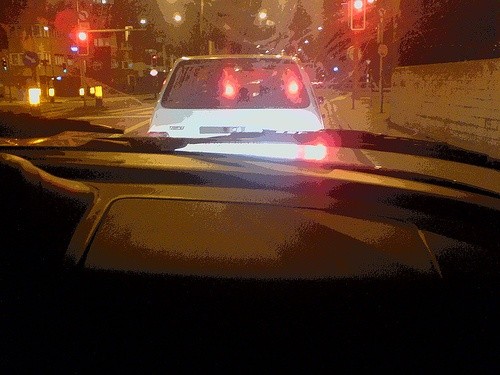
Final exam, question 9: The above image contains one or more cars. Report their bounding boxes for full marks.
[147,53,325,137]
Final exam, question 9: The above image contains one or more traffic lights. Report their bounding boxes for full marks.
[76,29,90,57]
[351,0,367,31]
[151,52,157,68]
[1,56,9,73]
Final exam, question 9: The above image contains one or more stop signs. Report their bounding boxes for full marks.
[76,8,90,22]
